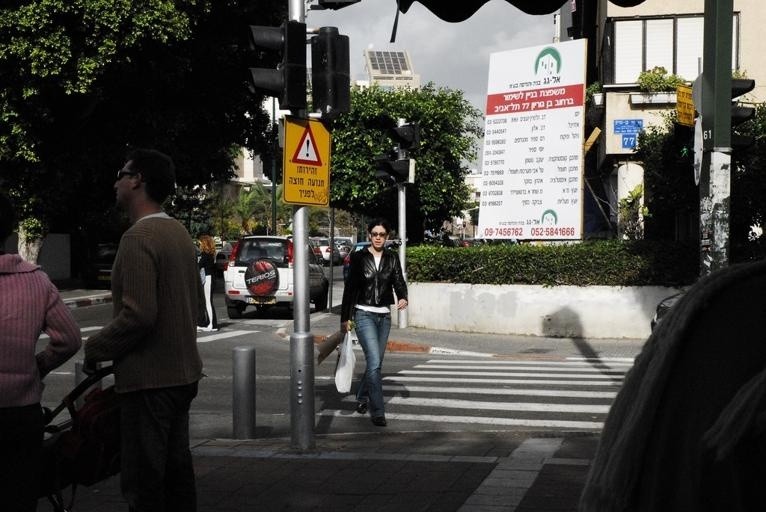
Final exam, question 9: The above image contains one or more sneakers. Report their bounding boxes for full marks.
[357,402,367,413]
[370,415,387,425]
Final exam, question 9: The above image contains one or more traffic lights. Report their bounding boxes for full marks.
[733,78,757,155]
[390,123,414,186]
[245,18,302,114]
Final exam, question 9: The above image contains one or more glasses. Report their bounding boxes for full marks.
[116,170,134,181]
[370,232,386,237]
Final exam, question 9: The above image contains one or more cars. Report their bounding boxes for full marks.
[211,230,358,272]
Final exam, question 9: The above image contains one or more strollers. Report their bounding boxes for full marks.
[35,359,120,509]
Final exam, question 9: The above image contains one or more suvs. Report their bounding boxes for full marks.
[222,230,332,318]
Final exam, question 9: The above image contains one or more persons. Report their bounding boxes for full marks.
[84,151,212,510]
[565,260,765,510]
[192,228,222,337]
[1,196,79,511]
[340,219,408,428]
